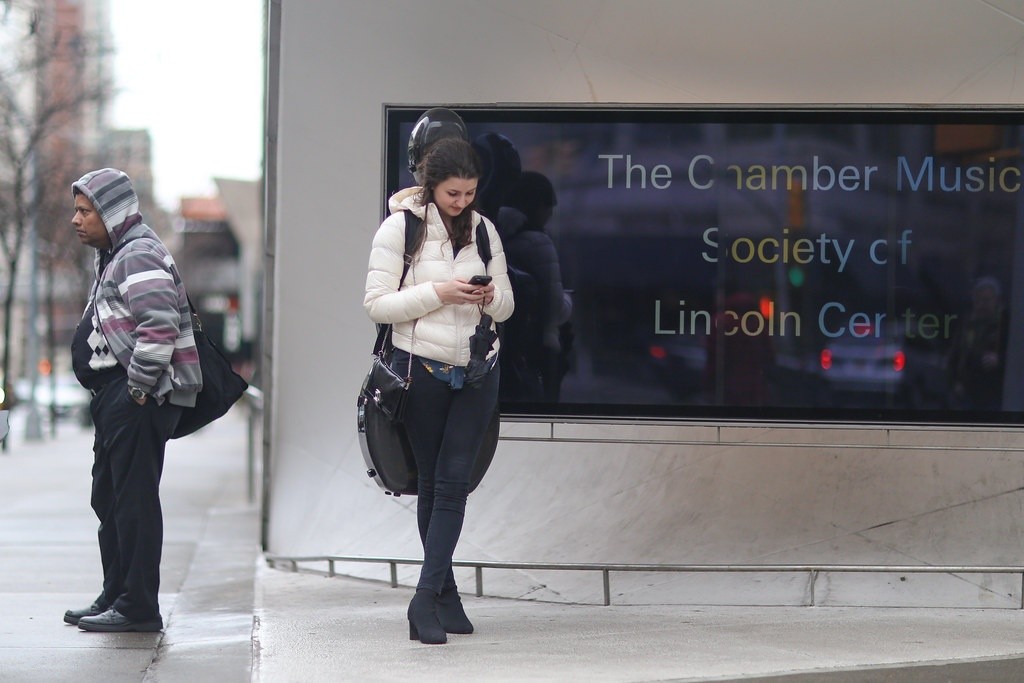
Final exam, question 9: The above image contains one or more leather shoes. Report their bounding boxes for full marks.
[64,603,163,632]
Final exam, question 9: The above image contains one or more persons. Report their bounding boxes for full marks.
[63,168,203,629]
[364,138,514,645]
[496,172,574,411]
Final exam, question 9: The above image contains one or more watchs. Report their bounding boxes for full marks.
[129,385,145,400]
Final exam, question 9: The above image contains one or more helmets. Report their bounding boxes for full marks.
[470,132,521,185]
[407,107,468,173]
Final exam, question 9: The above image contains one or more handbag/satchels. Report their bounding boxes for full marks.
[363,354,411,427]
[169,328,249,439]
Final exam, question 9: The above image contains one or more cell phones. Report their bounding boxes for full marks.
[466,276,492,287]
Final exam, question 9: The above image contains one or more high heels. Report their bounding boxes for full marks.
[407,591,474,644]
